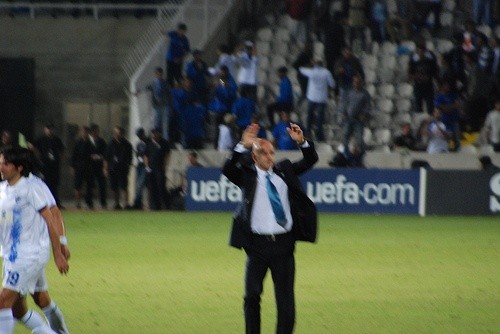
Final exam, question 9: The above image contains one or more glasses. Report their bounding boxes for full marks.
[261,152,276,156]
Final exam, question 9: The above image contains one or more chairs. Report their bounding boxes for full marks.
[0,0,500,159]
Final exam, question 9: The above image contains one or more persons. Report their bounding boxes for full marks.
[0,0,500,211]
[0,145,69,334]
[221,122,320,334]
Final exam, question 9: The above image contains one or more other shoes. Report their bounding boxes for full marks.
[58,205,66,211]
[114,205,133,210]
[74,203,80,208]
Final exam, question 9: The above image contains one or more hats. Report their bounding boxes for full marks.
[242,39,255,47]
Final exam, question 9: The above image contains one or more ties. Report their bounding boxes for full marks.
[265,172,287,228]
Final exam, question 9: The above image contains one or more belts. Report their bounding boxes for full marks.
[250,229,296,242]
